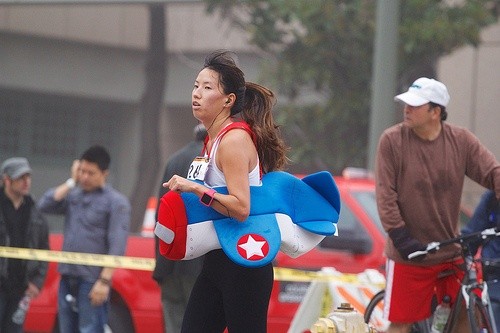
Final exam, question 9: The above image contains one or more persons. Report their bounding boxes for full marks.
[151,120,208,333]
[163,49,295,333]
[461,188,500,333]
[38,145,131,333]
[374,78,499,333]
[0,157,50,333]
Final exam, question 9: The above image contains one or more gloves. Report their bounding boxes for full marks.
[389,226,427,259]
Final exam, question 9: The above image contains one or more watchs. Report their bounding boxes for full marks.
[201,187,218,208]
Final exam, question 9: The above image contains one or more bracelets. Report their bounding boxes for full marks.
[67,178,75,189]
[97,275,111,287]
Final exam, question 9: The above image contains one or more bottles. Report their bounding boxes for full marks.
[12,296,30,325]
[431,295,452,333]
[65,294,79,312]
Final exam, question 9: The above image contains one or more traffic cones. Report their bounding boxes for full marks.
[141,196,158,238]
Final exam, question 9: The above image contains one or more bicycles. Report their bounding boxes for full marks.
[365,227,499,332]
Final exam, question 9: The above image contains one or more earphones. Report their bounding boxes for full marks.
[226,98,230,103]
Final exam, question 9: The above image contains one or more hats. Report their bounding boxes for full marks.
[393,76,450,106]
[1,157,32,179]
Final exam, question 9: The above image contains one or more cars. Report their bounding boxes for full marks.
[26,167,499,332]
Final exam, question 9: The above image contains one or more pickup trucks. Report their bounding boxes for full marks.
[22,166,476,331]
